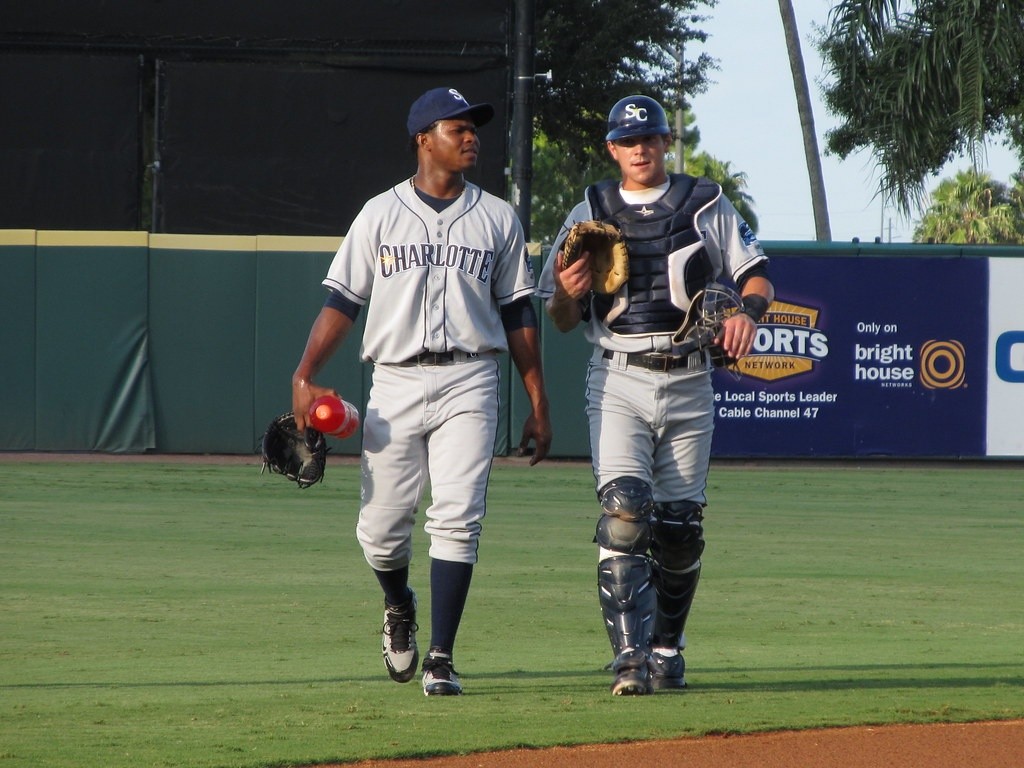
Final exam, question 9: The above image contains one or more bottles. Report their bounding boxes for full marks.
[309,396,359,439]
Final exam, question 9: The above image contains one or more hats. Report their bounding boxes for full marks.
[407,87,496,137]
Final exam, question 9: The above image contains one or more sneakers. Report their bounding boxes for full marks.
[382,585,419,683]
[611,664,652,695]
[421,645,462,696]
[652,678,687,689]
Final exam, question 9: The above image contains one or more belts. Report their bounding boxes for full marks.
[406,350,477,367]
[603,350,705,371]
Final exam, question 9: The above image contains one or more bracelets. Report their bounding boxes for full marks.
[735,293,769,325]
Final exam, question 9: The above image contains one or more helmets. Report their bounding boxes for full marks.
[605,96,669,141]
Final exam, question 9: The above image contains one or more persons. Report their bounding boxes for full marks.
[534,95,773,695]
[291,88,553,695]
